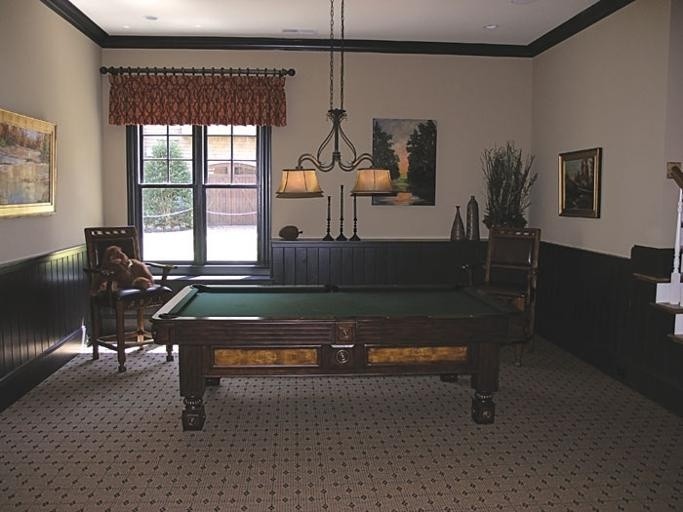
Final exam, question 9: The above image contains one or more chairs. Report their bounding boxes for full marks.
[84,226,175,372]
[480,227,541,367]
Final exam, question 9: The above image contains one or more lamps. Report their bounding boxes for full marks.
[275,0,401,199]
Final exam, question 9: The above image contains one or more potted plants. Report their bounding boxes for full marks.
[478,138,539,234]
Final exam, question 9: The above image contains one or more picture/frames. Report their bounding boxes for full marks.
[0,108,58,219]
[557,146,603,219]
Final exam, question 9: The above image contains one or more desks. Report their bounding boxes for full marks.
[150,283,511,431]
